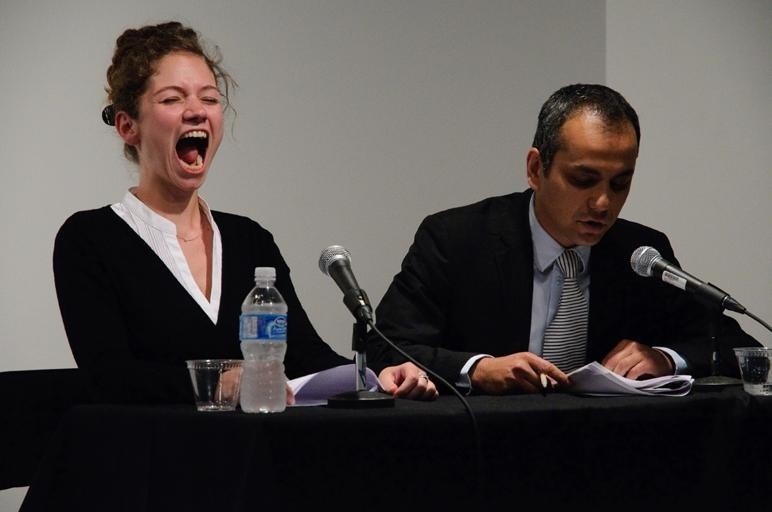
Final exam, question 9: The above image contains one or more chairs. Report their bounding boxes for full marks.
[0,366,90,495]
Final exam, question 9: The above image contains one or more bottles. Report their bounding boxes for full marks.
[237,267,290,414]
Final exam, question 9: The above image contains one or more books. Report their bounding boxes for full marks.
[563,361,695,398]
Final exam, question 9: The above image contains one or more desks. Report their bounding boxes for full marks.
[24,376,772,512]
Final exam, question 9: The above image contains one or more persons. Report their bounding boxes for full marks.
[364,82,763,397]
[52,21,441,407]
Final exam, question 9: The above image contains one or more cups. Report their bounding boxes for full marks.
[184,358,244,414]
[732,346,772,396]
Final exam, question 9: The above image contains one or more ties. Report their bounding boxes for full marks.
[539,250,588,383]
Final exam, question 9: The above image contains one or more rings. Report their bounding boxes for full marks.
[418,373,429,382]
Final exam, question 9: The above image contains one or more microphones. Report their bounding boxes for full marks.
[631,246,746,315]
[319,246,373,323]
